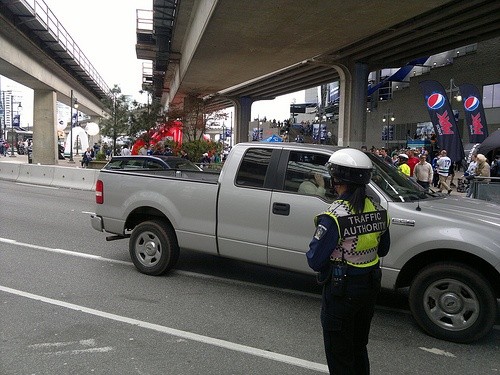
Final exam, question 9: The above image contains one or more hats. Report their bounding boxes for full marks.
[477,154,487,161]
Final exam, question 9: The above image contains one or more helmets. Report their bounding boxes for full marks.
[398,154,409,161]
[327,148,373,184]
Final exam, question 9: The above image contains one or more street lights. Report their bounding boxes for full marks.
[8,95,23,157]
[315,104,326,142]
[445,78,462,107]
[68,89,78,161]
[383,113,396,154]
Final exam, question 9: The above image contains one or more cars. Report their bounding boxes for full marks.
[101,155,201,171]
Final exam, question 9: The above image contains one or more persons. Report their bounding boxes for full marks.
[81,148,93,168]
[306,147,391,375]
[3,140,10,157]
[298,168,326,199]
[94,141,191,159]
[360,146,492,198]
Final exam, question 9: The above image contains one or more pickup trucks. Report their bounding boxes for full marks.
[91,142,500,344]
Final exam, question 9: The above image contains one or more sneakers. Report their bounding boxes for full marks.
[447,189,452,194]
[437,190,442,193]
